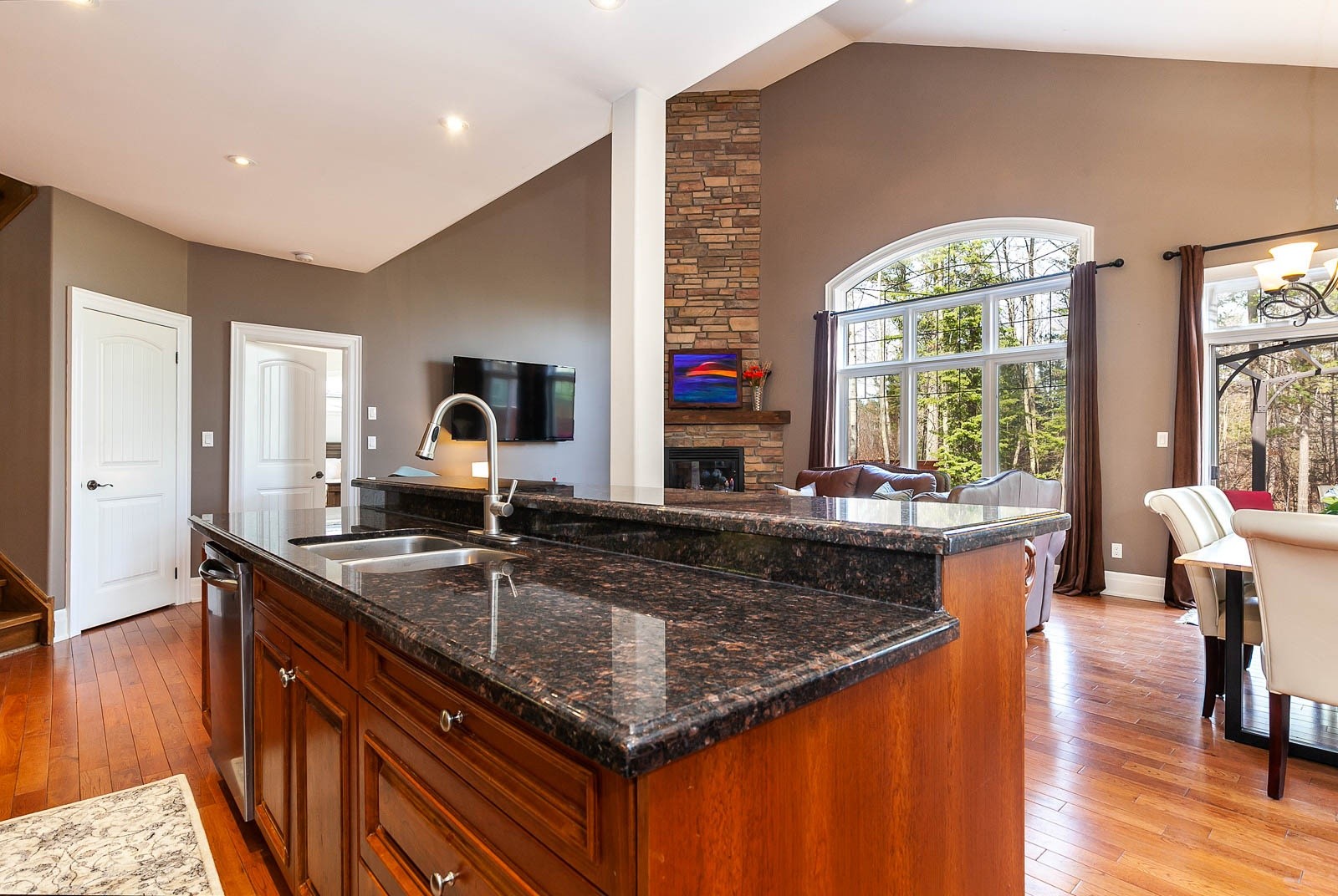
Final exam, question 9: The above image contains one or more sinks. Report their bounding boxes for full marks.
[288,525,470,564]
[340,545,535,576]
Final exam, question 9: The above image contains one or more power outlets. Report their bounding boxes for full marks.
[1110,543,1123,558]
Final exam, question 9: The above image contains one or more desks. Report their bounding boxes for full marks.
[1174,532,1338,767]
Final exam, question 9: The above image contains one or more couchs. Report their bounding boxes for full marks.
[795,464,1066,632]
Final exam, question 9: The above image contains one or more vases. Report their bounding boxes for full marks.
[751,385,763,412]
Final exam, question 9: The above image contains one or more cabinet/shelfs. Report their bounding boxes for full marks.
[248,567,644,896]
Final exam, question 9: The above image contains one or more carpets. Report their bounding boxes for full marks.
[0,772,223,896]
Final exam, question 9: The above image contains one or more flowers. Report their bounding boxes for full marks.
[745,360,773,386]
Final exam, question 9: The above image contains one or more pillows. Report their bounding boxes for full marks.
[773,483,817,500]
[871,480,914,503]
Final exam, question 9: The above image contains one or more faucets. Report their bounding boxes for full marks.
[412,392,522,543]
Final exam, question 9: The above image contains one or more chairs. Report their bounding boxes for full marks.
[1147,486,1338,800]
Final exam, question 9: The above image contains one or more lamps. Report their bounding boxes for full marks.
[1254,240,1338,327]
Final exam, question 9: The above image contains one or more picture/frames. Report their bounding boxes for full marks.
[669,349,742,409]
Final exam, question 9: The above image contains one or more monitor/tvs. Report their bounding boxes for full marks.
[452,356,576,441]
